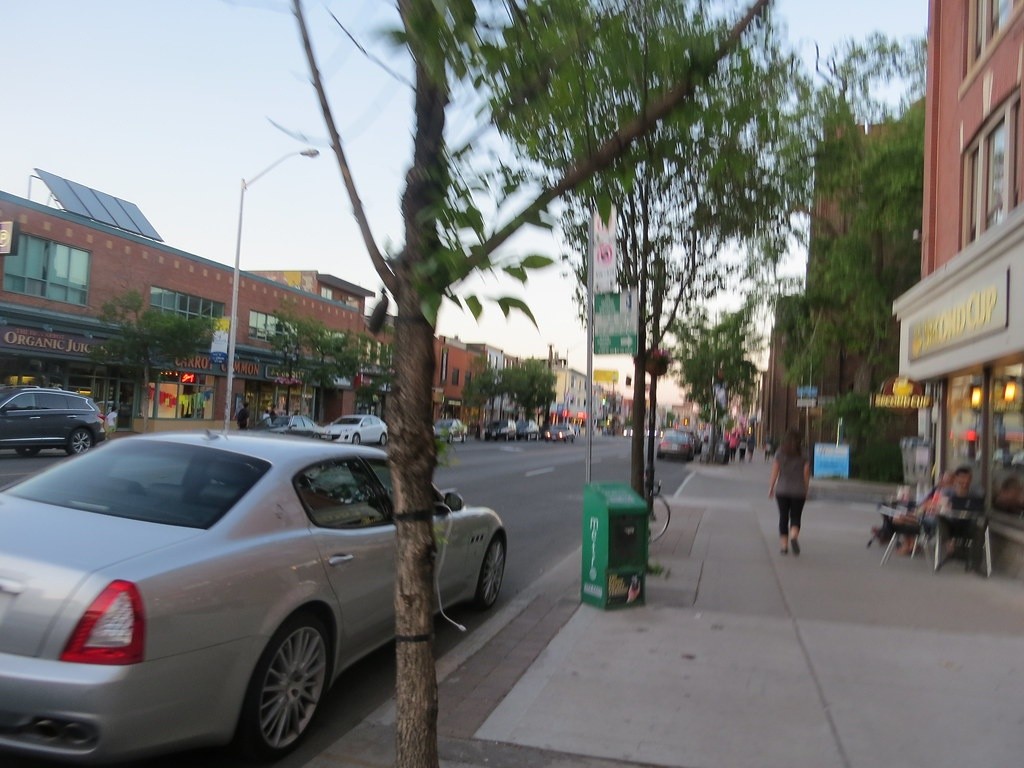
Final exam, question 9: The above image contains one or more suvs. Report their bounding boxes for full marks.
[0,385,107,456]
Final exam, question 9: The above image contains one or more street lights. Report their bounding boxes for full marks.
[224,149,321,430]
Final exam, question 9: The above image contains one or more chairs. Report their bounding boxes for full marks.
[104,477,241,508]
[877,497,993,577]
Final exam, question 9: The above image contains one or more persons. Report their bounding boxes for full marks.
[237,402,250,430]
[104,406,118,439]
[730,432,754,463]
[764,437,771,461]
[263,405,276,419]
[575,423,580,438]
[768,428,811,556]
[871,466,984,566]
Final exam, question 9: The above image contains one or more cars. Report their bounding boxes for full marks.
[515,420,540,442]
[1,432,509,768]
[245,415,327,442]
[433,419,468,443]
[657,429,694,461]
[485,420,517,442]
[545,424,576,443]
[320,415,388,446]
[692,433,702,454]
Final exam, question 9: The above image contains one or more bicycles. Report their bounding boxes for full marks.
[646,468,670,542]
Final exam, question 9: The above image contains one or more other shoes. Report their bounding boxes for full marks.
[748,460,752,464]
[781,547,787,555]
[739,461,746,464]
[791,539,801,556]
